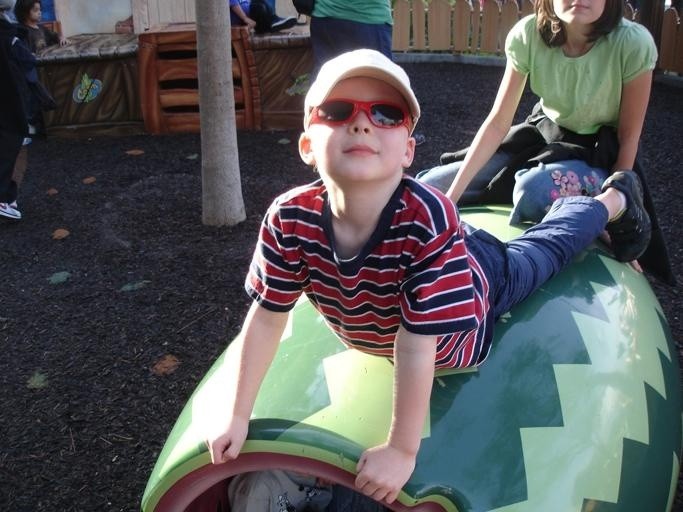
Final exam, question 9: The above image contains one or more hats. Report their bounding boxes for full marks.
[304,49,420,138]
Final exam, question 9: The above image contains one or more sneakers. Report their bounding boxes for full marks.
[601,168,653,264]
[1,203,22,220]
[272,16,297,31]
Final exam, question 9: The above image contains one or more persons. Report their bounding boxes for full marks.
[290,0,426,148]
[0,0,72,222]
[229,0,298,35]
[412,0,660,272]
[200,48,653,504]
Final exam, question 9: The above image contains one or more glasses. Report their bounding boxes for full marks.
[307,97,413,130]
